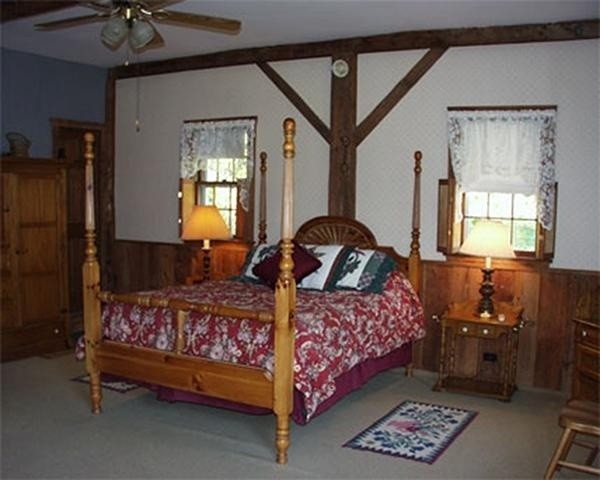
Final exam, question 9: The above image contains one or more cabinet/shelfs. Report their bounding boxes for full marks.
[0,160,74,363]
[570,317,600,404]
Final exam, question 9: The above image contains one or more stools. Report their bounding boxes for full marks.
[543,415,600,480]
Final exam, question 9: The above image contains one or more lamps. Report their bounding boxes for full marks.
[459,221,517,319]
[100,18,155,133]
[182,206,231,282]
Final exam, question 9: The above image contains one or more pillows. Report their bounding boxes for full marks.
[333,247,400,294]
[252,240,322,292]
[231,244,353,293]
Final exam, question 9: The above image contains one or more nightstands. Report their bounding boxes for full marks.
[431,308,525,403]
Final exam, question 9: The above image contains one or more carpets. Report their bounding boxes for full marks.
[342,399,479,465]
[70,373,141,394]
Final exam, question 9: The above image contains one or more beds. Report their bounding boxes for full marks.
[82,118,422,465]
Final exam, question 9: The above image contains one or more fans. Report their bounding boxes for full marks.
[32,0,242,54]
